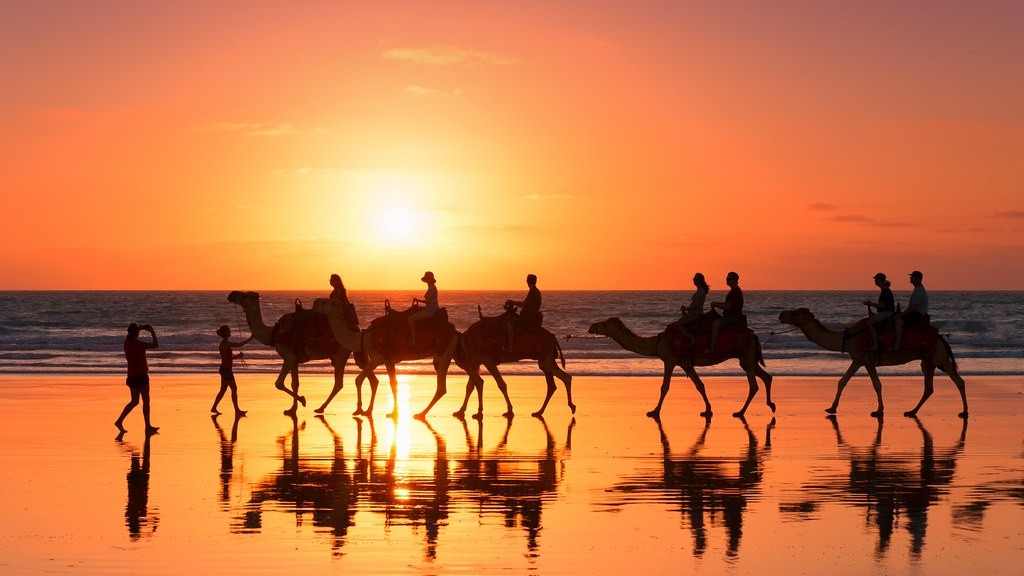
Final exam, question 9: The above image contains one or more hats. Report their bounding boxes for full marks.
[873,273,887,279]
[422,272,437,283]
[909,271,923,278]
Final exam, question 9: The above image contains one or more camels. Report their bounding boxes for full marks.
[587,308,776,417]
[226,291,577,421]
[779,304,969,420]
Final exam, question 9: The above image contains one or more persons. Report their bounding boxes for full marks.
[863,273,895,350]
[308,274,348,341]
[212,325,254,416]
[885,271,928,351]
[115,323,159,433]
[677,273,709,347]
[703,272,744,353]
[403,271,439,346]
[503,275,542,354]
